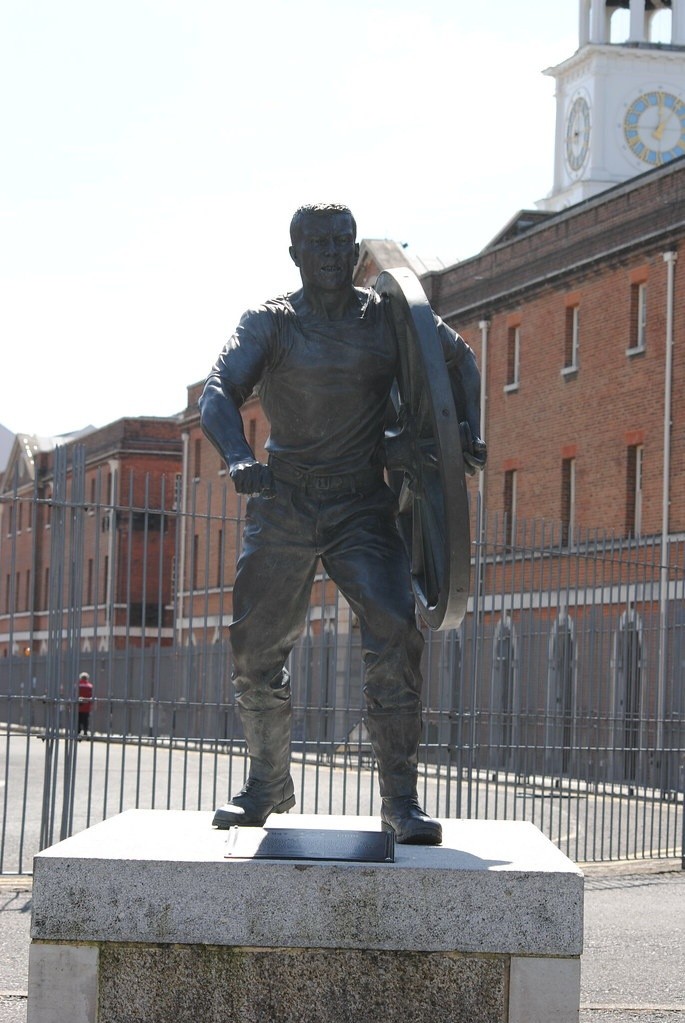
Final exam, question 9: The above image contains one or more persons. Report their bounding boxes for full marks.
[74,672,93,736]
[199,202,485,844]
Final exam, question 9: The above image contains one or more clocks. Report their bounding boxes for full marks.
[613,79,685,171]
[562,84,593,182]
